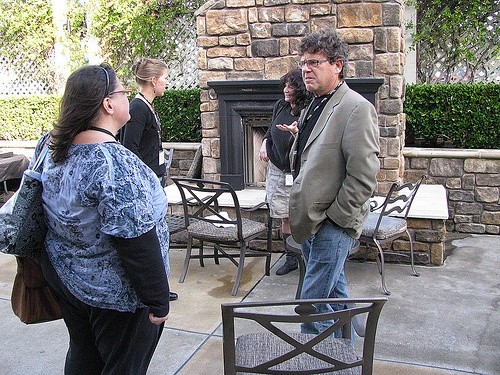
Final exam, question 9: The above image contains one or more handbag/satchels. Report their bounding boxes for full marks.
[11,259,63,324]
[0,142,47,258]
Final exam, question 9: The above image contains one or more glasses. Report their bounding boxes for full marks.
[105,88,132,98]
[298,58,339,69]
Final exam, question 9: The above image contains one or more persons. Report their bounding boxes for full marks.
[260,69,314,274]
[29,64,170,375]
[117,57,178,300]
[289,30,379,347]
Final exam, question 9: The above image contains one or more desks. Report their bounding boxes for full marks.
[0,153,30,203]
[286,234,360,300]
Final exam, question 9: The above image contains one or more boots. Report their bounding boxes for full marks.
[276,233,298,275]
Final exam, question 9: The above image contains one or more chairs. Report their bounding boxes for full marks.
[221,297,388,375]
[361,176,424,295]
[167,177,273,297]
